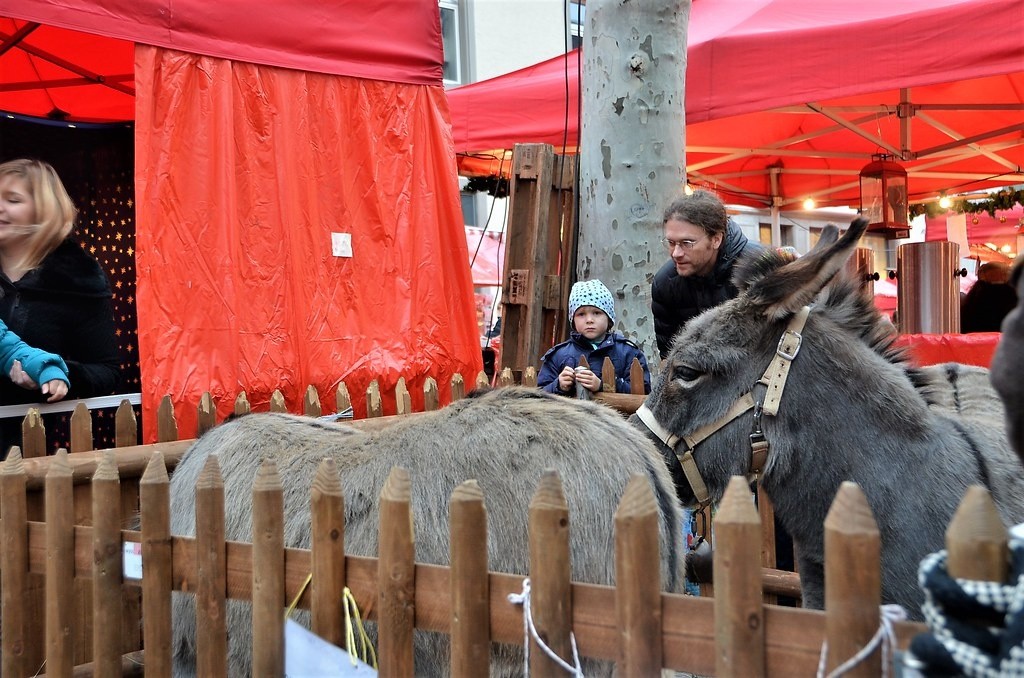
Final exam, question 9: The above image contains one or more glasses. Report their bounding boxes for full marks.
[661,233,710,250]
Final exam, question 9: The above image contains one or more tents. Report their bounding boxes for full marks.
[445,1,1024,209]
[0,0,487,446]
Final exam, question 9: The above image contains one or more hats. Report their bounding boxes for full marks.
[568,279,615,332]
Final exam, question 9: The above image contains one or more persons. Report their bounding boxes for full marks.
[960,261,1019,335]
[652,190,767,359]
[537,279,651,395]
[0,158,122,402]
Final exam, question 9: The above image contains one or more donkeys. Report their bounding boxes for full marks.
[170,384,687,678]
[625,213,1023,621]
